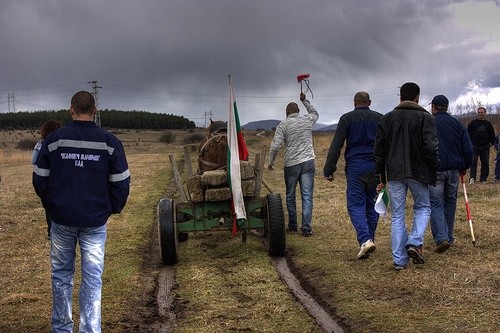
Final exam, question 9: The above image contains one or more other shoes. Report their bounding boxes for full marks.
[469,178,474,186]
[481,181,487,186]
[302,229,313,237]
[287,227,298,233]
[433,242,454,254]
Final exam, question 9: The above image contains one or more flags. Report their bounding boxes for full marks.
[227,75,249,238]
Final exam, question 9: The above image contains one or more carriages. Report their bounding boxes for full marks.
[157,117,286,265]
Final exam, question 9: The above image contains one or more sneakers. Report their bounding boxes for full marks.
[391,264,406,269]
[408,246,426,265]
[356,239,376,260]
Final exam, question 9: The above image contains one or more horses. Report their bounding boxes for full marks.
[197,118,229,176]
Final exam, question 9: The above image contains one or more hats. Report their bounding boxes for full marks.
[429,95,449,107]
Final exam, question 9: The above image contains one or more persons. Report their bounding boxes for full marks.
[493,128,500,182]
[323,91,386,259]
[426,95,473,254]
[31,119,64,240]
[465,107,495,185]
[33,91,130,333]
[269,93,320,237]
[372,83,440,269]
